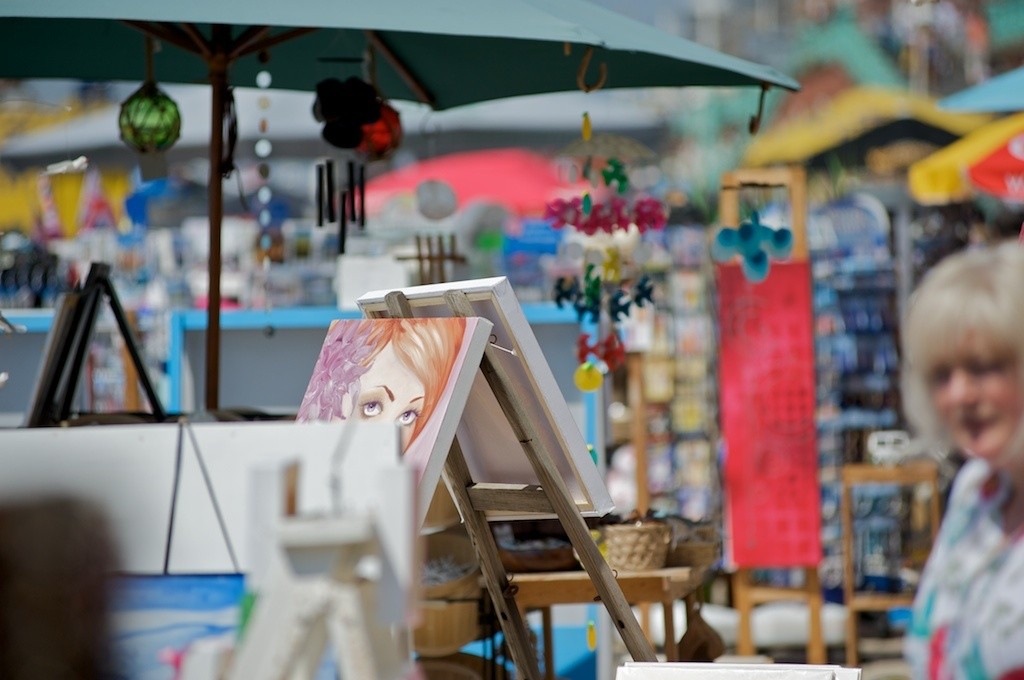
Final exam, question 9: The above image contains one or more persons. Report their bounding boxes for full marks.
[898,241,1024,680]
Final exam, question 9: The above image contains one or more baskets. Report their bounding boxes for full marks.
[595,522,670,573]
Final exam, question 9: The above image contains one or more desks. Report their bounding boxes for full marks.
[504,562,707,680]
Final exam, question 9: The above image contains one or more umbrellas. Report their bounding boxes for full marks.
[0,0,804,411]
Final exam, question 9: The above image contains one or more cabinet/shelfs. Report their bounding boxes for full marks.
[715,461,941,679]
